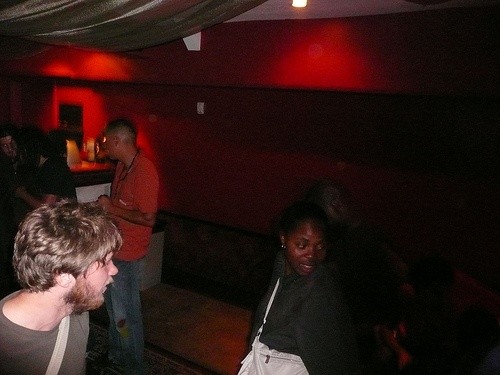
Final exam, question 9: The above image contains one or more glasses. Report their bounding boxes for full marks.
[1,143,17,150]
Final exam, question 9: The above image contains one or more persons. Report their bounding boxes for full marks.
[248,177,417,375]
[1,125,78,300]
[95,117,160,375]
[0,198,124,375]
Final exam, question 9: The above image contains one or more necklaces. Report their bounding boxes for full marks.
[113,147,140,203]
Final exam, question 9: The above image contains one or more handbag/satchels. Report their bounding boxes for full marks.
[238,278,310,375]
